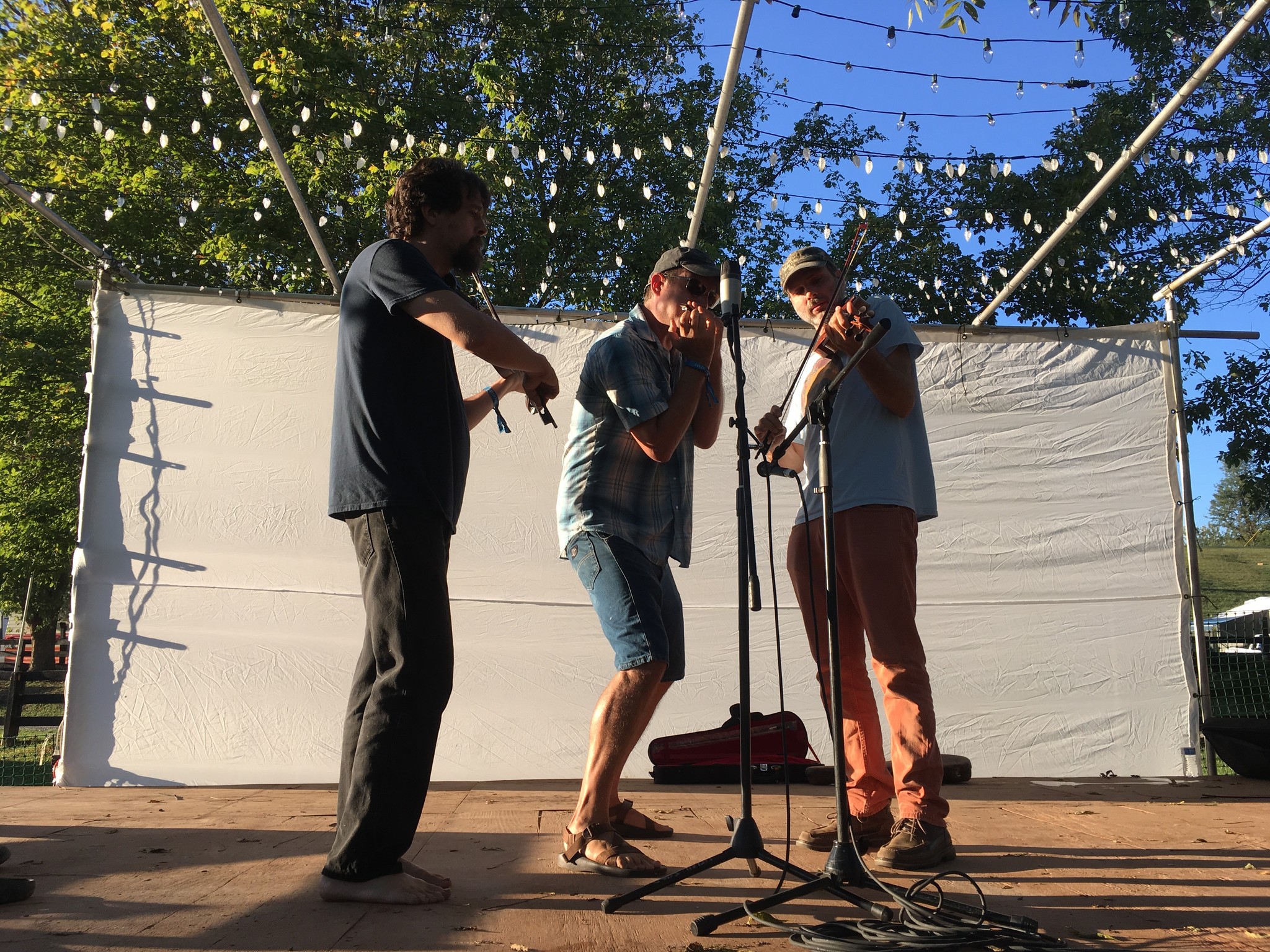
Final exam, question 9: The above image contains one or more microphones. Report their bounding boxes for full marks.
[719,260,741,346]
[756,460,798,478]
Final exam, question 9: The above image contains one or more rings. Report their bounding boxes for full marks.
[713,314,716,319]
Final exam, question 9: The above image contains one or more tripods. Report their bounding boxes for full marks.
[600,317,1047,933]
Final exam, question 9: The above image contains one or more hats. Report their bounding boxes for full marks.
[648,246,720,285]
[779,246,838,294]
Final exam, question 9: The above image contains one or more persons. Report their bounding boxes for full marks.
[322,157,559,905]
[559,248,724,877]
[747,247,959,868]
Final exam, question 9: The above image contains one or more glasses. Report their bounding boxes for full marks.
[643,274,720,309]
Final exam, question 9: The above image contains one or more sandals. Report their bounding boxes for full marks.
[558,823,667,878]
[609,798,674,839]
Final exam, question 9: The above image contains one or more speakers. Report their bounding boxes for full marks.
[1191,609,1270,779]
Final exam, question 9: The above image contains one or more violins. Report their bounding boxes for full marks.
[429,267,557,430]
[812,294,875,360]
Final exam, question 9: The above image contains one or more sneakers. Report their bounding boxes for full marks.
[874,818,956,871]
[796,805,895,852]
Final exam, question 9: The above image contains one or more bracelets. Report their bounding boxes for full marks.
[482,386,511,433]
[685,359,719,408]
